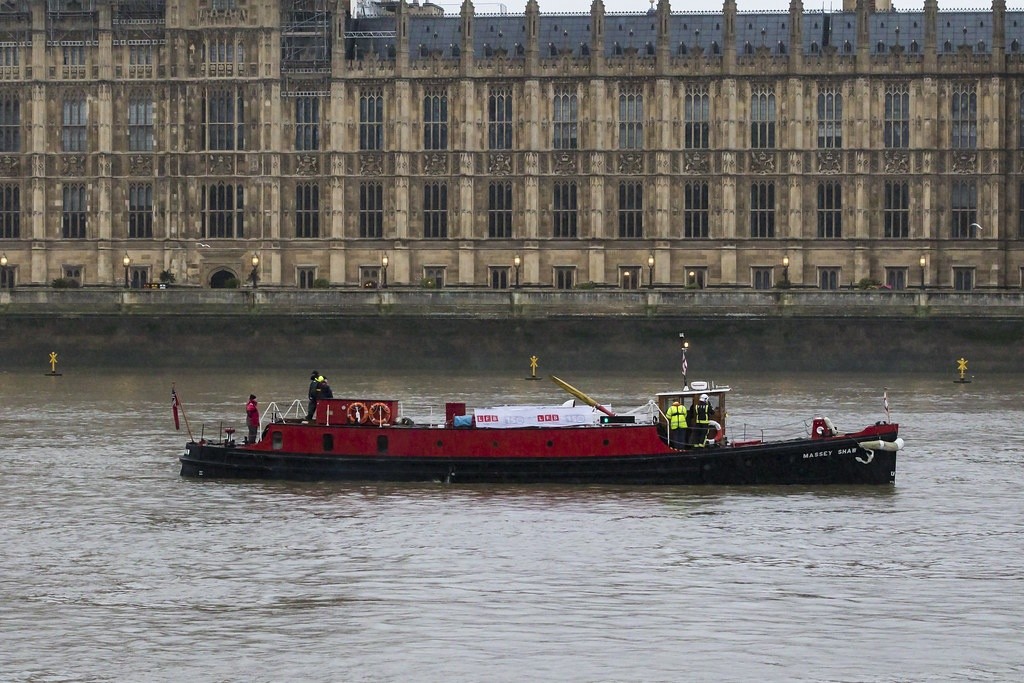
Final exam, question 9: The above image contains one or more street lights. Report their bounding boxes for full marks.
[782,255,789,289]
[382,252,391,288]
[919,254,926,290]
[647,254,656,289]
[0,251,8,289]
[513,253,521,289]
[123,251,131,289]
[251,253,260,288]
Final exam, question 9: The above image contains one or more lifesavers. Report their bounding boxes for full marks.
[368,401,391,427]
[705,419,723,443]
[346,401,370,426]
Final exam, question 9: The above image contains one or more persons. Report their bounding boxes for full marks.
[245,394,259,443]
[311,376,333,399]
[694,393,715,449]
[666,399,688,449]
[304,372,328,419]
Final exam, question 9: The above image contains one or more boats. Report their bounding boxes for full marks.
[179,373,905,487]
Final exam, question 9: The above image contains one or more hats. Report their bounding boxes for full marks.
[250,395,256,400]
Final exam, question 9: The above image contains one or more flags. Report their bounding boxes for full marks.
[170,389,180,430]
[682,354,688,376]
[882,393,889,412]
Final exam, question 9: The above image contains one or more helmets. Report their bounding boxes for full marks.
[699,393,710,404]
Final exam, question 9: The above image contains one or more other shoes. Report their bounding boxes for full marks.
[699,443,706,448]
[305,416,313,421]
[693,444,699,448]
